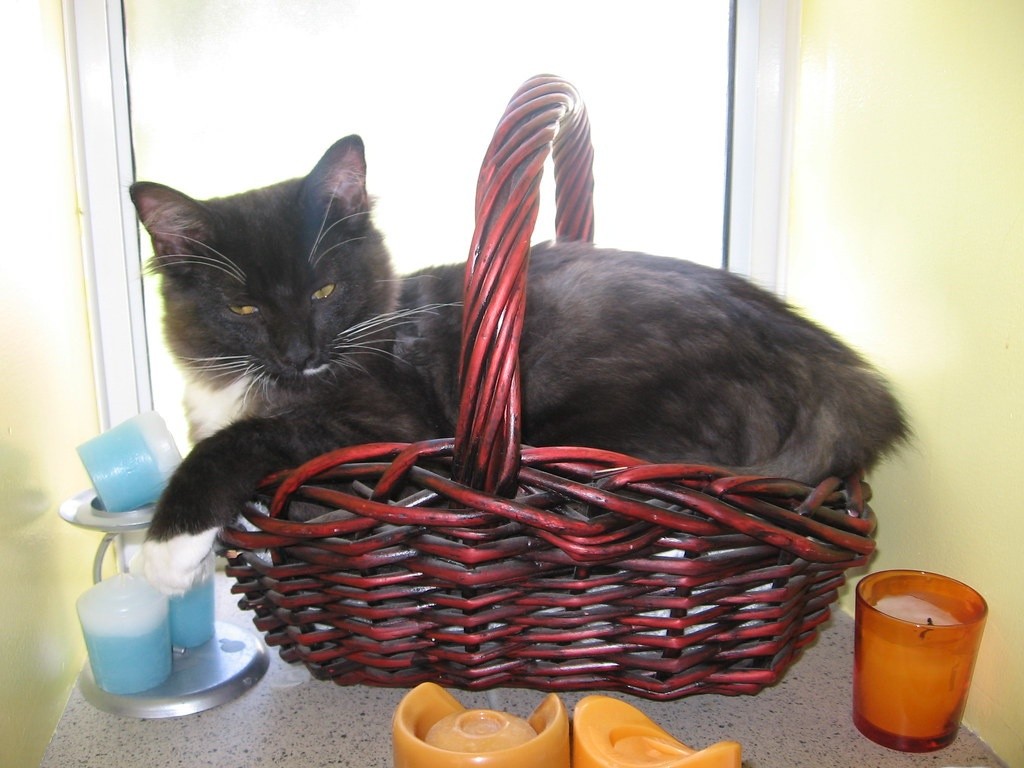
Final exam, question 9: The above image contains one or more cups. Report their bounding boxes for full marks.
[853,571,988,753]
[168,547,215,649]
[78,587,172,693]
[77,412,184,512]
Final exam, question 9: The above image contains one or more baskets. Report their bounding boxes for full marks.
[214,71,880,701]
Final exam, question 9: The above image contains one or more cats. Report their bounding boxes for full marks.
[127,134,919,599]
